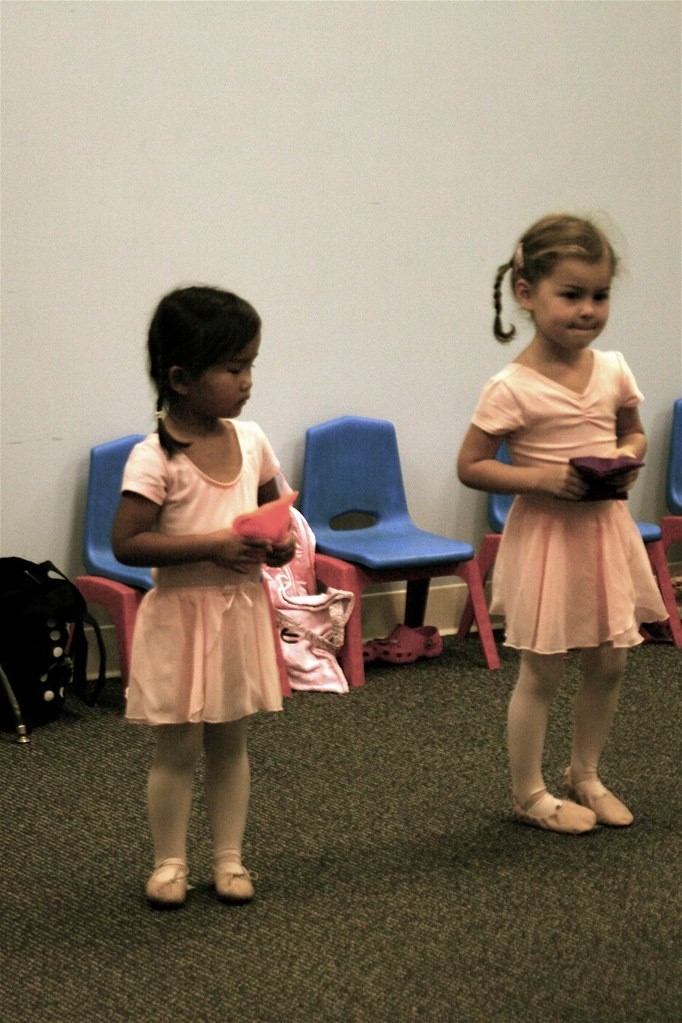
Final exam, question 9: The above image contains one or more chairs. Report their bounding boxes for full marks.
[61,431,156,694]
[658,397,682,553]
[454,436,682,652]
[297,414,502,692]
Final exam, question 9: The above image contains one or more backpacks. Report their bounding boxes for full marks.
[0,556,107,733]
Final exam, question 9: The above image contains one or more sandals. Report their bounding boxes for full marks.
[340,637,382,662]
[369,623,443,662]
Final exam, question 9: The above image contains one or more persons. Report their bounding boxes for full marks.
[455,217,671,833]
[111,284,296,903]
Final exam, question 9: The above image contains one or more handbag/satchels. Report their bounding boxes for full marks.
[262,570,356,694]
